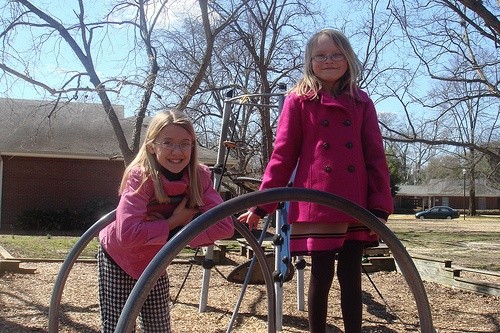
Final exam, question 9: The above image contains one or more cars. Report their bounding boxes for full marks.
[415,205,460,220]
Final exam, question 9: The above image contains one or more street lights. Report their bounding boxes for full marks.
[462,167,467,220]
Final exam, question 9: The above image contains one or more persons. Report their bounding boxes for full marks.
[236,28,395,333]
[96,108,234,333]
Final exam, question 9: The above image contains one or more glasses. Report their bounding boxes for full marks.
[153,140,193,149]
[311,53,347,62]
